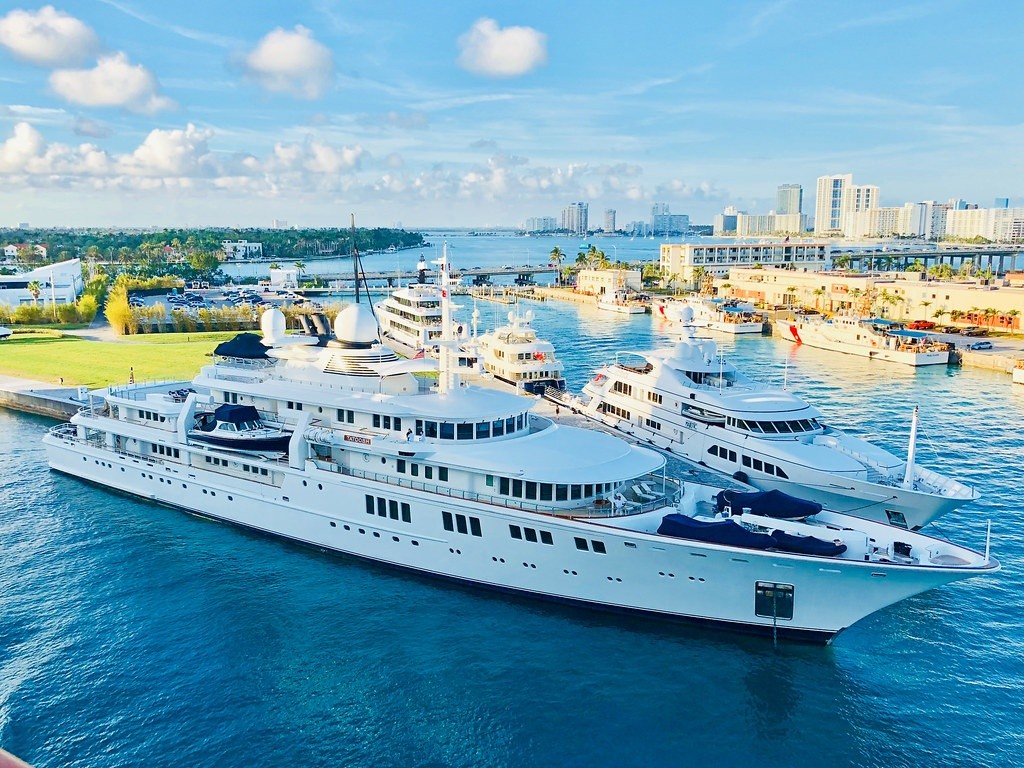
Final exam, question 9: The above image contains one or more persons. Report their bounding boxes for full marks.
[168,387,197,402]
[613,496,626,515]
[406,428,425,443]
[872,324,951,353]
[556,405,561,420]
[723,311,762,323]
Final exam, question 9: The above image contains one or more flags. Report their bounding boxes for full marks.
[413,348,424,359]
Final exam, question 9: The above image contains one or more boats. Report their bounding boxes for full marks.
[186,403,293,461]
[545,336,985,532]
[595,288,646,315]
[38,303,1004,643]
[371,255,473,360]
[776,309,951,367]
[458,303,568,392]
[651,296,693,322]
[716,489,823,522]
[704,307,764,334]
[655,512,848,559]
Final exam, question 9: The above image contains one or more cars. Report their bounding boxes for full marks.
[908,319,989,338]
[970,341,992,350]
[129,287,263,310]
